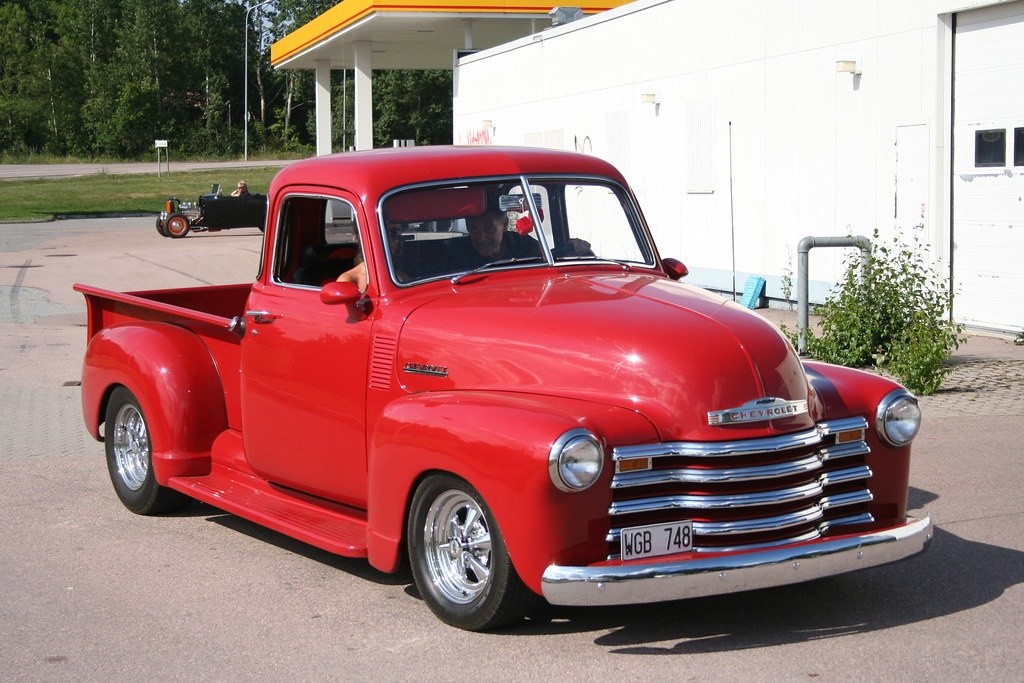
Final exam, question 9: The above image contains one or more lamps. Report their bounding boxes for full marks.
[836,60,863,74]
[483,119,497,127]
[641,92,661,104]
[549,6,580,27]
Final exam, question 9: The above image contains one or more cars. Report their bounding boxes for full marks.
[155,182,267,239]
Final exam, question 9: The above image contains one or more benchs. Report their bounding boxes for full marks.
[318,244,462,286]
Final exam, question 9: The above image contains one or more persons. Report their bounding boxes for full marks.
[320,223,413,291]
[231,181,250,196]
[436,197,591,273]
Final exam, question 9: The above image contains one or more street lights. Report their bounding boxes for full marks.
[244,0,274,162]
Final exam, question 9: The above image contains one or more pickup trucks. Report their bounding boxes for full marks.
[68,144,938,635]
